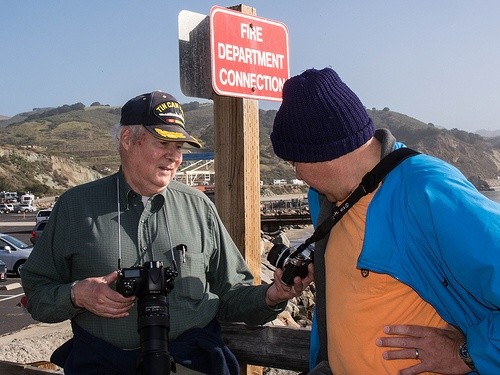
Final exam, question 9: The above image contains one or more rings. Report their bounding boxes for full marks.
[415,348,418,359]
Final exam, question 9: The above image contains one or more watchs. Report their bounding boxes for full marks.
[459,337,477,372]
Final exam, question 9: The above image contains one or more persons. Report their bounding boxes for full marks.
[270,65,500,375]
[20,89,314,375]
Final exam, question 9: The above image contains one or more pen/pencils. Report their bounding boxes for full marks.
[183,246,185,262]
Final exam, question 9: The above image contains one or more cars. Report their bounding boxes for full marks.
[18,204,37,212]
[0,233,34,278]
[0,203,15,214]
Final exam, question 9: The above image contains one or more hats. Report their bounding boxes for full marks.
[120,90,202,149]
[271,67,376,162]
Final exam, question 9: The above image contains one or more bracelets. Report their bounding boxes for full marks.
[70,287,78,308]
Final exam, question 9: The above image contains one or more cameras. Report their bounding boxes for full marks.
[267,241,315,287]
[115,260,178,375]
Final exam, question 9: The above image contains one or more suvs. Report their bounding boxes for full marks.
[30,208,53,244]
